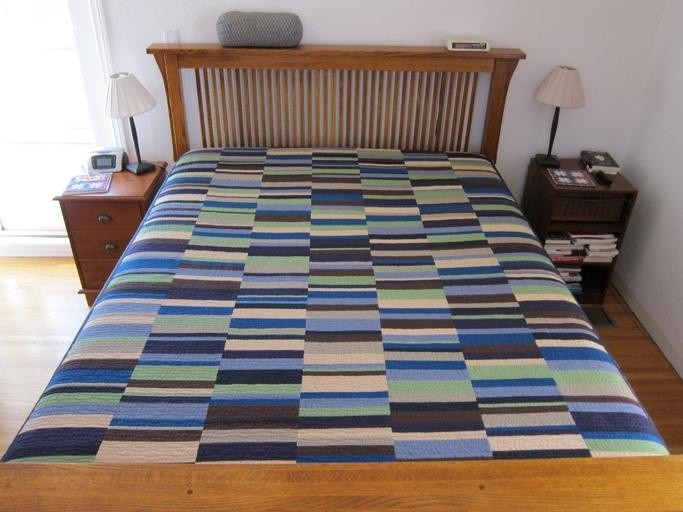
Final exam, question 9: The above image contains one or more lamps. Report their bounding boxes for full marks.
[532,63,586,170]
[103,70,158,177]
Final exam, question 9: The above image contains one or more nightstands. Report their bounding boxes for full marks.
[521,157,638,310]
[52,160,169,309]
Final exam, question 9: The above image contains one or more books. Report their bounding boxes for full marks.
[546,150,620,187]
[63,172,113,194]
[543,232,619,293]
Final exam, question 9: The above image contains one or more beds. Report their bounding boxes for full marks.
[0,43,683,511]
